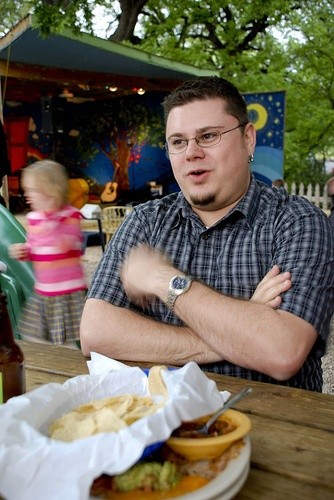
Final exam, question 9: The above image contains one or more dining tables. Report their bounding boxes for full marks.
[14,336,334,500]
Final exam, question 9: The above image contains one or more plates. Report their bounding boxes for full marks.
[90,430,252,500]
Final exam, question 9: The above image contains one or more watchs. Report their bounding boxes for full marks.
[167,274,196,311]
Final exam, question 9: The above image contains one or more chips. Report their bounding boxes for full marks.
[49,393,157,442]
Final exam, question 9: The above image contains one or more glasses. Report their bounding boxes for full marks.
[162,125,244,155]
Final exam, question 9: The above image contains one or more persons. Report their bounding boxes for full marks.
[272,179,283,187]
[80,76,334,393]
[9,160,88,350]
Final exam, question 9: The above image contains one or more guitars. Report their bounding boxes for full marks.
[101,162,120,203]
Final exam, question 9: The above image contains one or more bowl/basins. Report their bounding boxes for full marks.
[164,408,251,461]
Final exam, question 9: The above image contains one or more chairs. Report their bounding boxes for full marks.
[101,205,134,244]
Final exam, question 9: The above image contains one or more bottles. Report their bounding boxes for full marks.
[0,291,26,406]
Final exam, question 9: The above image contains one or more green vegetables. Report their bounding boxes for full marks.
[111,461,182,491]
